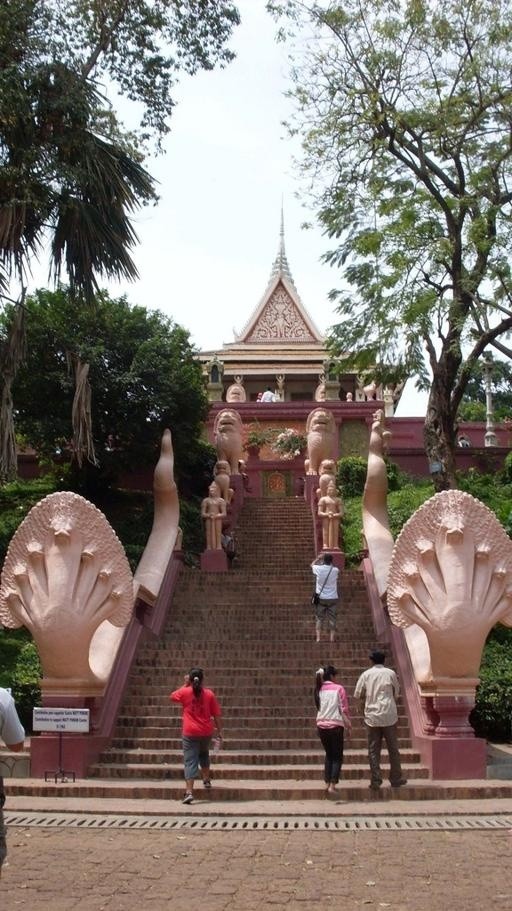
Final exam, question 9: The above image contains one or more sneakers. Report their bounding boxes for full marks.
[182,792,194,803]
[203,779,211,788]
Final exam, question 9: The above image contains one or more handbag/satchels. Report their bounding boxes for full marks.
[312,592,319,604]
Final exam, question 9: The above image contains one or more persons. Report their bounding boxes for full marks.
[353,652,407,790]
[310,553,340,642]
[170,669,223,804]
[317,480,344,549]
[260,386,277,402]
[200,481,227,550]
[457,437,470,448]
[256,393,262,402]
[0,687,26,869]
[314,666,352,794]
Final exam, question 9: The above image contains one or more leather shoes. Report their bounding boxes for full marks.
[391,779,408,787]
[368,781,380,789]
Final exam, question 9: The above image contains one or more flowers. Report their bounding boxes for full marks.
[270,427,308,462]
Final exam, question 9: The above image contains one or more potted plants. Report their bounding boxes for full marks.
[243,429,263,457]
